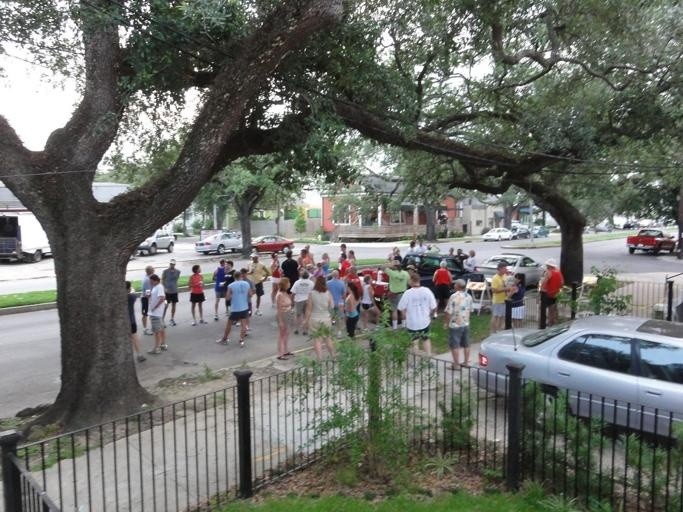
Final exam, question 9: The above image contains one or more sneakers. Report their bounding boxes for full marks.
[137,309,262,363]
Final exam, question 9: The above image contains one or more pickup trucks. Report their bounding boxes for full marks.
[351,252,484,303]
[623,228,674,254]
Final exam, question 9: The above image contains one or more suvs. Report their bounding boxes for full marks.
[135,229,175,255]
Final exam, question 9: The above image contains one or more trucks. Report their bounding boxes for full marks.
[0,212,52,262]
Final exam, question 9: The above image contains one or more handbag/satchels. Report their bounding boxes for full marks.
[362,303,369,310]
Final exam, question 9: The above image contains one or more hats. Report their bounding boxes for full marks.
[250,253,256,257]
[545,258,557,268]
[169,258,176,264]
[392,260,401,265]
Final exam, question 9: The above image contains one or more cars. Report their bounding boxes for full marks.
[194,231,242,254]
[475,252,544,292]
[238,234,295,256]
[471,314,682,438]
[480,224,549,242]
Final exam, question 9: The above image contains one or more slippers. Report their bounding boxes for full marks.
[447,364,461,370]
[462,361,472,368]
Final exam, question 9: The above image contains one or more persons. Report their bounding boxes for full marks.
[445,279,472,371]
[126,281,147,363]
[213,240,477,360]
[161,258,180,327]
[540,258,561,329]
[490,263,511,333]
[188,265,207,326]
[141,266,154,335]
[147,274,168,355]
[505,273,526,330]
